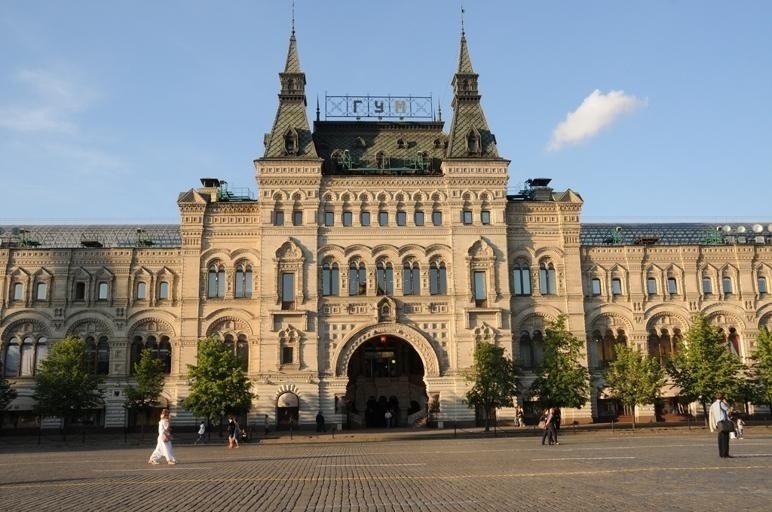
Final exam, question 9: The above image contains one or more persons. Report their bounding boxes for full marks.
[554,405,562,429]
[384,408,393,428]
[544,407,560,444]
[538,408,555,445]
[316,409,327,433]
[736,415,745,439]
[514,415,519,427]
[728,406,737,437]
[517,411,525,428]
[230,417,243,448]
[227,416,236,448]
[147,412,176,464]
[708,392,735,458]
[193,421,210,446]
[148,407,178,464]
[264,413,271,435]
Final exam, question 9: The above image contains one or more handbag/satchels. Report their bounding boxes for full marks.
[715,420,734,433]
